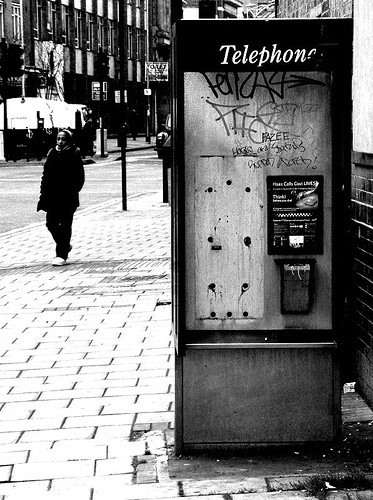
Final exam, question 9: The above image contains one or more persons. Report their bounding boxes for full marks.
[36,130,84,268]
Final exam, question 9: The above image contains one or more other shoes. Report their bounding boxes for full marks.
[52,257,66,265]
[68,245,72,253]
